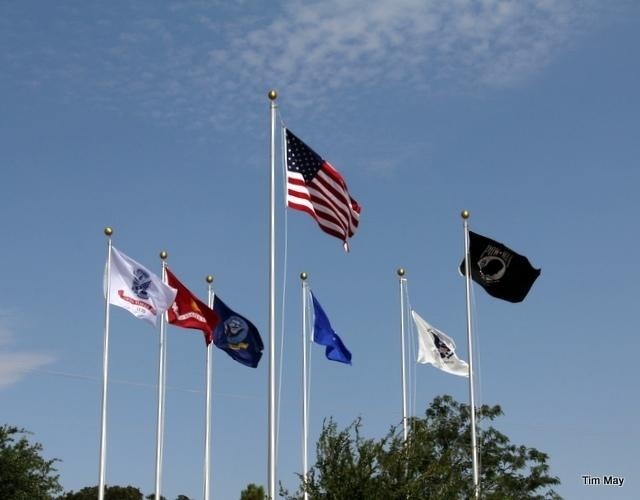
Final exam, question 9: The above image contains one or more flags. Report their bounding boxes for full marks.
[164,265,219,346]
[309,291,353,366]
[283,129,362,253]
[458,230,542,303]
[101,246,175,325]
[209,292,264,368]
[411,310,470,378]
